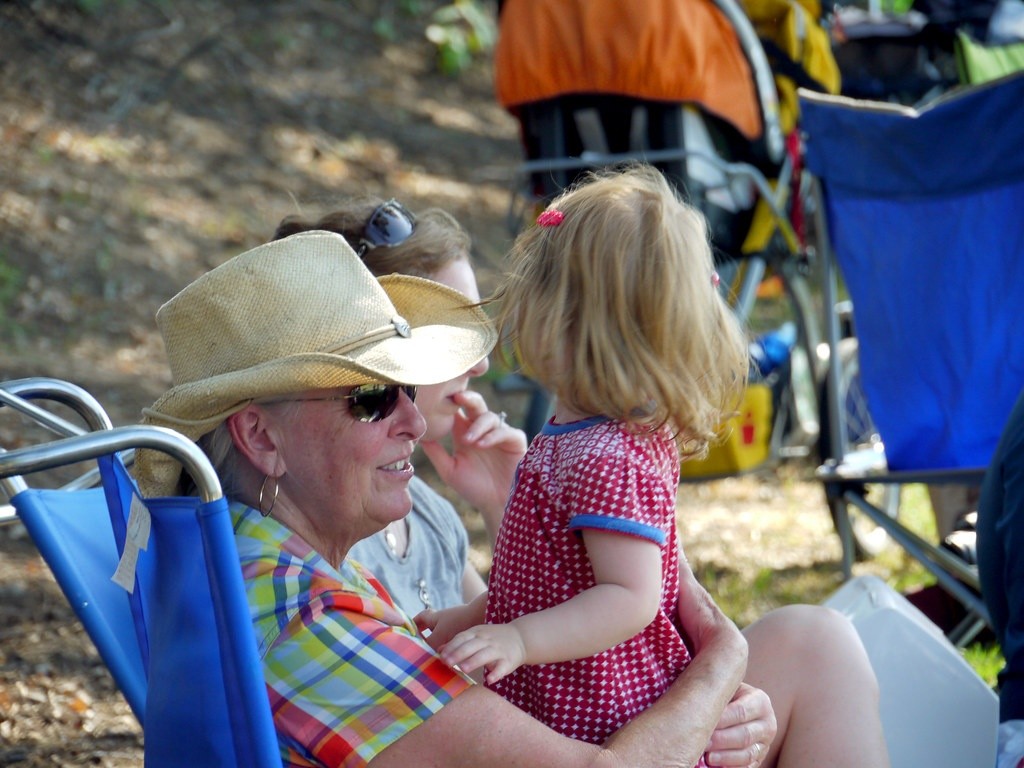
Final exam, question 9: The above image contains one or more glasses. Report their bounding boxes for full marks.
[254,382,420,424]
[356,196,417,258]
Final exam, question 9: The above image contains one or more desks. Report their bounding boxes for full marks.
[829,20,954,101]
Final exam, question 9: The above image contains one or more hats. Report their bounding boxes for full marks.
[130,230,498,501]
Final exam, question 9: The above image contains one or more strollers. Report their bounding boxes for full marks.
[494,3,848,521]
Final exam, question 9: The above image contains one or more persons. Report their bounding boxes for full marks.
[136,162,888,768]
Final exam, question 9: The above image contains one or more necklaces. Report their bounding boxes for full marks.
[383,525,397,556]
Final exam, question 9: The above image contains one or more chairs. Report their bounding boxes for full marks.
[473,0,825,451]
[792,65,1024,636]
[0,378,298,768]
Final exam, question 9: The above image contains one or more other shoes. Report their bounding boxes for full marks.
[995,718,1024,768]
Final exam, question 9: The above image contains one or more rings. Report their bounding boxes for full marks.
[498,411,507,428]
[755,742,760,761]
